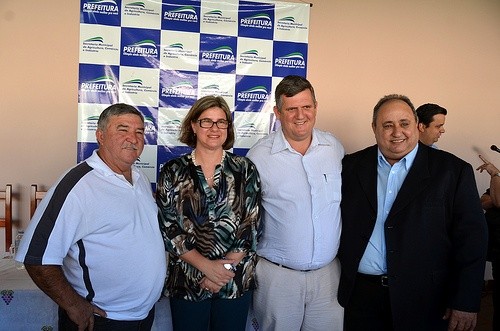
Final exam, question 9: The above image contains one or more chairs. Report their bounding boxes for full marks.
[0,185,47,252]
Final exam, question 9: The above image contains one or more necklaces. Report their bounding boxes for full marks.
[192,148,226,180]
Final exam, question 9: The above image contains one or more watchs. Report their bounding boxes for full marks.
[223,257,236,271]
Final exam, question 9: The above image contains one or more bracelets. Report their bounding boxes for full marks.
[492,173,500,177]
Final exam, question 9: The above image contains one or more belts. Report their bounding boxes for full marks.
[262,257,311,272]
[357,273,389,287]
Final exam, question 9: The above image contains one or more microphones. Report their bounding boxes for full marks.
[490,145,500,153]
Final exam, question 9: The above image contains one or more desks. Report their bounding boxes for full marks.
[0,252,262,331]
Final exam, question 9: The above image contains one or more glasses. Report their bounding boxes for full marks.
[197,118,231,129]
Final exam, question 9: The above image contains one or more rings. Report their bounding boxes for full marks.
[205,288,208,291]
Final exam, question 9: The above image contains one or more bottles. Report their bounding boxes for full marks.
[15,228,25,270]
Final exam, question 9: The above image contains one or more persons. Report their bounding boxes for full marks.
[14,104,168,331]
[337,94,487,331]
[415,103,447,147]
[477,155,500,281]
[156,95,263,331]
[245,76,345,331]
